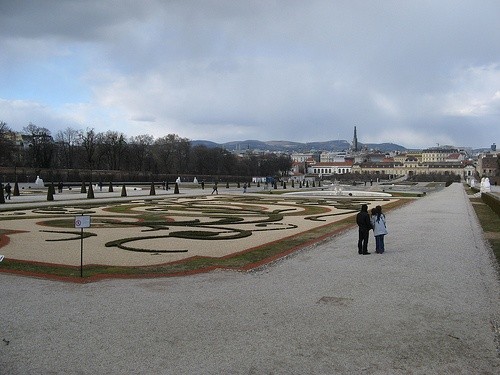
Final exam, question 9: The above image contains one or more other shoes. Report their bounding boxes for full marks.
[375,251,382,254]
[363,252,371,255]
[358,252,363,254]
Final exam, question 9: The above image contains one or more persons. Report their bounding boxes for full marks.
[211,183,218,195]
[356,205,374,255]
[243,184,247,192]
[371,206,387,254]
[4,182,12,200]
[57,183,63,194]
[201,181,204,189]
[162,179,169,190]
